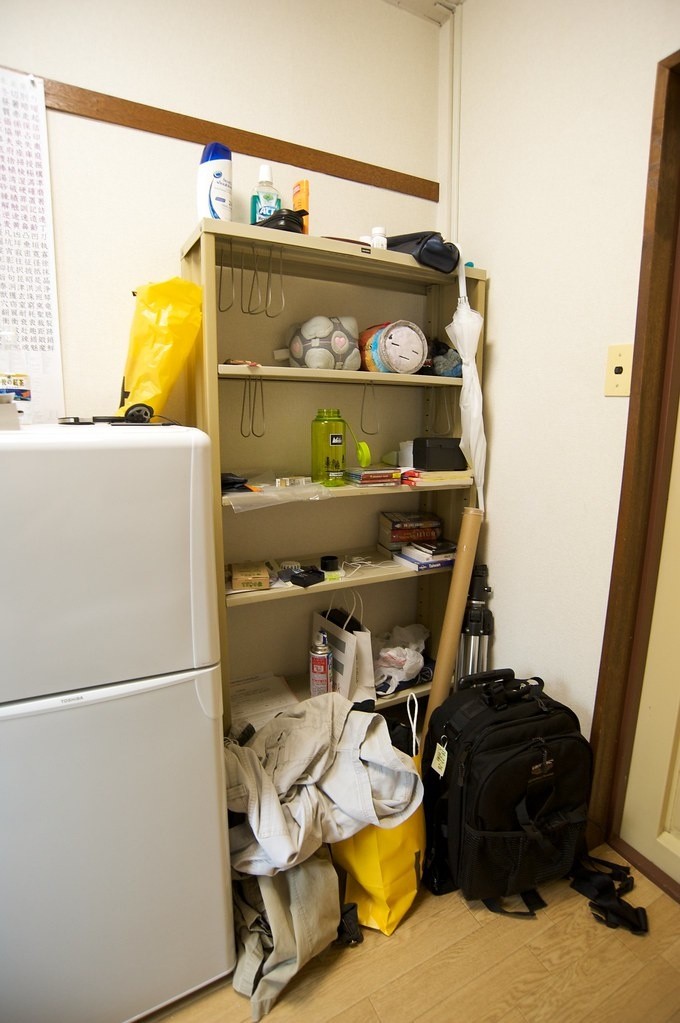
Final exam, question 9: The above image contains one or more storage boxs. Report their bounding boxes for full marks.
[412,436,468,471]
[232,562,270,590]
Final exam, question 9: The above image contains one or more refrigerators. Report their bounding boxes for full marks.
[0,425,236,1023]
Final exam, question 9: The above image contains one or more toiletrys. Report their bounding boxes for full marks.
[289,178,311,235]
[194,141,235,223]
[249,164,284,230]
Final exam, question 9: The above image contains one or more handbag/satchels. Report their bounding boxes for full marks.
[117,276,202,422]
[330,693,424,937]
[310,587,378,705]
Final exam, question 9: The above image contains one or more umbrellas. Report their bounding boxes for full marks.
[444,242,488,513]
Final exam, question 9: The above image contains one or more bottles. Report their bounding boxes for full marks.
[372,227,388,250]
[250,164,281,225]
[308,631,333,698]
[196,143,233,223]
[311,408,372,487]
[291,179,309,235]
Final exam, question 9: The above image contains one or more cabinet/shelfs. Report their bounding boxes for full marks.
[182,218,487,739]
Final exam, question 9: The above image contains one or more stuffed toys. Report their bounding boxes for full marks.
[414,335,463,378]
[285,313,363,371]
[357,319,429,374]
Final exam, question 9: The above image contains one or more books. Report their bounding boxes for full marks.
[379,509,443,529]
[342,464,474,487]
[378,536,412,551]
[378,522,444,542]
[226,671,300,735]
[392,553,455,572]
[410,537,457,555]
[401,544,457,562]
[376,543,402,560]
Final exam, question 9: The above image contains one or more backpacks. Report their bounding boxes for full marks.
[421,675,650,937]
